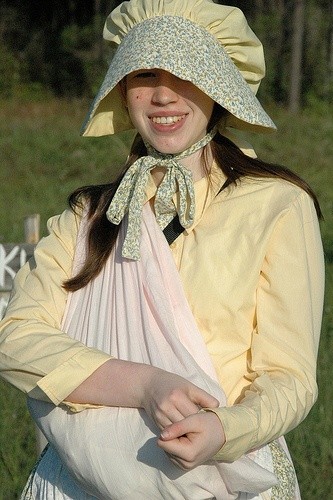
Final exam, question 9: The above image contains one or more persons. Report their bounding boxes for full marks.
[0,0,332,500]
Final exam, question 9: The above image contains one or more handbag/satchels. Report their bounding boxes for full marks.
[26,201,278,499]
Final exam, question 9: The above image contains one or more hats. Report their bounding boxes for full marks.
[80,1,278,261]
